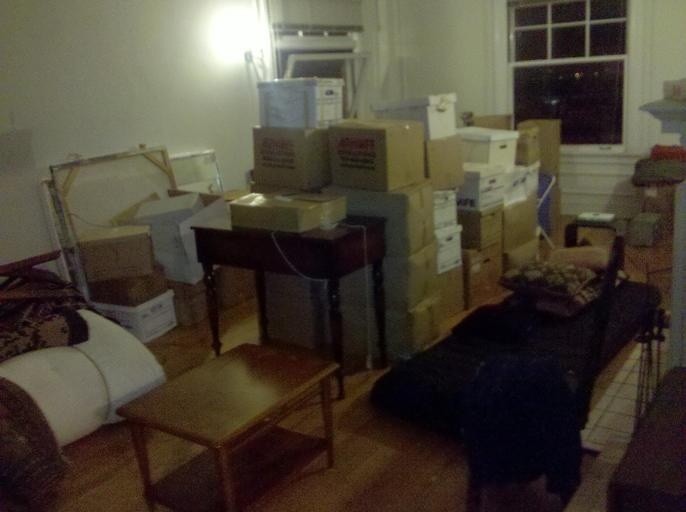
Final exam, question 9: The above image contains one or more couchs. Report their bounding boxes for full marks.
[0,250,167,454]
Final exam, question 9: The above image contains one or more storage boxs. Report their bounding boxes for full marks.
[86,265,171,306]
[456,157,506,210]
[499,160,541,208]
[328,115,425,190]
[433,222,465,274]
[231,187,350,235]
[323,293,444,364]
[420,136,466,190]
[255,70,348,131]
[459,118,521,173]
[431,185,458,230]
[370,91,456,140]
[89,291,181,343]
[513,124,540,168]
[321,179,436,254]
[320,239,440,309]
[258,269,325,348]
[247,120,332,188]
[438,269,467,323]
[640,186,679,239]
[629,213,663,248]
[521,116,563,176]
[73,218,156,284]
[501,198,541,255]
[458,243,504,309]
[504,242,544,282]
[535,176,563,240]
[457,200,506,251]
[134,192,216,275]
[208,184,264,203]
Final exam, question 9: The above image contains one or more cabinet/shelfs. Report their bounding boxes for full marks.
[590,357,686,512]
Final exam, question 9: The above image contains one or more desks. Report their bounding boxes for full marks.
[192,196,389,402]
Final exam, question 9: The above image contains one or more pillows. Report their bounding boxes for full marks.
[548,247,611,271]
[500,260,598,301]
[529,269,629,317]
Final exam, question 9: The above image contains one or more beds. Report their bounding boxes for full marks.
[369,278,663,464]
